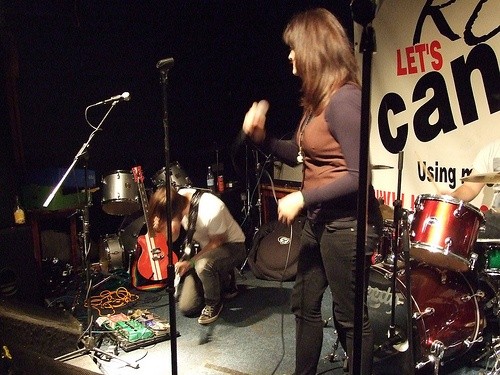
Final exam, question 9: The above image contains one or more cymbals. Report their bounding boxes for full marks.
[369,164,395,168]
[459,172,500,184]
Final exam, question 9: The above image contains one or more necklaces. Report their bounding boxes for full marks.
[297,106,313,162]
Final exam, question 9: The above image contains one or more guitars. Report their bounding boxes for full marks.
[130,166,178,281]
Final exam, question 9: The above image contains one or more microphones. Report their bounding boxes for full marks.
[103,92,130,104]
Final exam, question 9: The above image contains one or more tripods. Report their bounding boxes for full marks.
[41,100,139,369]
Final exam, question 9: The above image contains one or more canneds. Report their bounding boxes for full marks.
[217,175,224,191]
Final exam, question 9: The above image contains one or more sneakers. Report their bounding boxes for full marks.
[197,302,223,324]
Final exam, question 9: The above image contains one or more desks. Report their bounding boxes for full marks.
[26,205,82,291]
[260,179,302,226]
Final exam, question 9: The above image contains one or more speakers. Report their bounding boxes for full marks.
[274,158,305,183]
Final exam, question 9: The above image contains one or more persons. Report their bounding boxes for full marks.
[436,142,500,209]
[148,186,247,325]
[242,8,383,375]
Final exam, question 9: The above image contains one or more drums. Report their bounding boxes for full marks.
[480,245,500,276]
[404,194,487,272]
[98,237,126,275]
[332,259,485,375]
[99,169,142,216]
[151,161,193,190]
[377,219,406,262]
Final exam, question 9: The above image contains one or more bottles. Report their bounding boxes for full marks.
[11,194,27,226]
[206,167,214,188]
